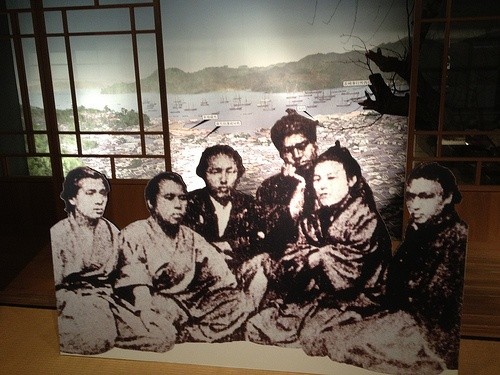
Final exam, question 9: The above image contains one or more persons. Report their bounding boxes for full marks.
[255,109,318,232]
[184,145,275,310]
[50,165,177,355]
[321,164,468,375]
[120,172,253,344]
[247,140,392,357]
